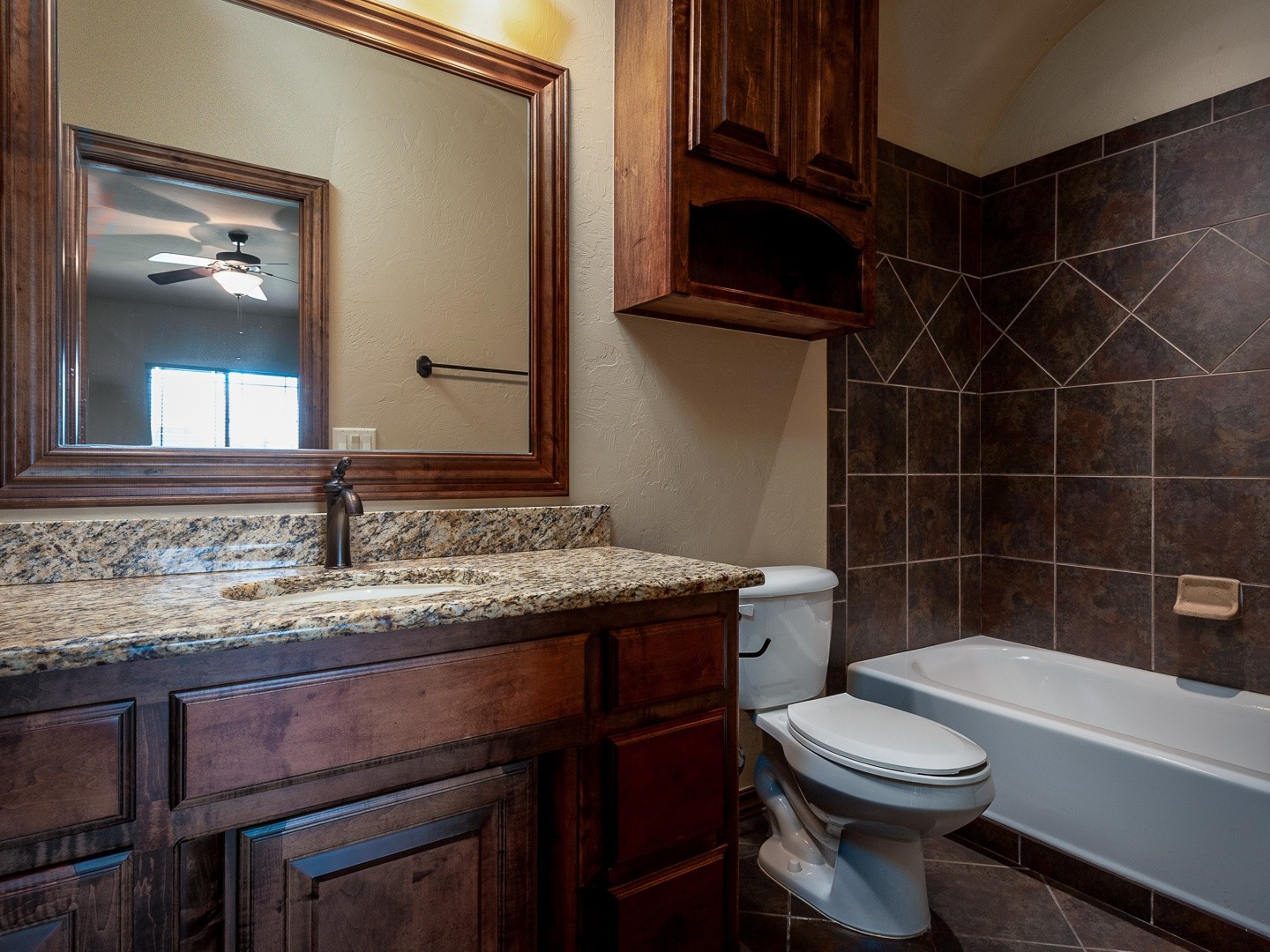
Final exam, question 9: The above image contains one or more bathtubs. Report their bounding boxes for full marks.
[845,634,1270,952]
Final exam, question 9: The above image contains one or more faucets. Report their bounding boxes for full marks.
[322,456,364,571]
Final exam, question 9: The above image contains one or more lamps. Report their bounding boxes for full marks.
[212,270,262,364]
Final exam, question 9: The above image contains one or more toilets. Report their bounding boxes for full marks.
[738,565,997,943]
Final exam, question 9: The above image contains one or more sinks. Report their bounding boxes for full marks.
[218,567,507,604]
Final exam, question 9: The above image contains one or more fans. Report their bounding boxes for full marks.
[147,234,299,285]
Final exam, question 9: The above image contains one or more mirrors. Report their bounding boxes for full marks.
[61,123,329,449]
[0,0,572,509]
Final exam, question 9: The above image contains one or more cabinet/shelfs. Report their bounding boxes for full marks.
[0,592,740,952]
[612,0,881,342]
